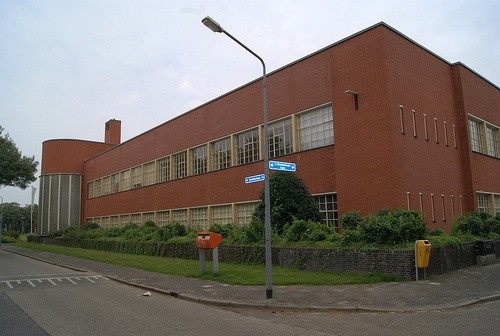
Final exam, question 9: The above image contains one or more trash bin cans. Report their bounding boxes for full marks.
[414,239,432,282]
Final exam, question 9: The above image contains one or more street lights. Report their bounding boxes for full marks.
[202,16,273,299]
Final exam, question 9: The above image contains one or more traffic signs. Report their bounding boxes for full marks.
[245,174,265,184]
[269,161,296,172]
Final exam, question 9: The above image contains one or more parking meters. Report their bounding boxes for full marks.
[414,240,432,285]
